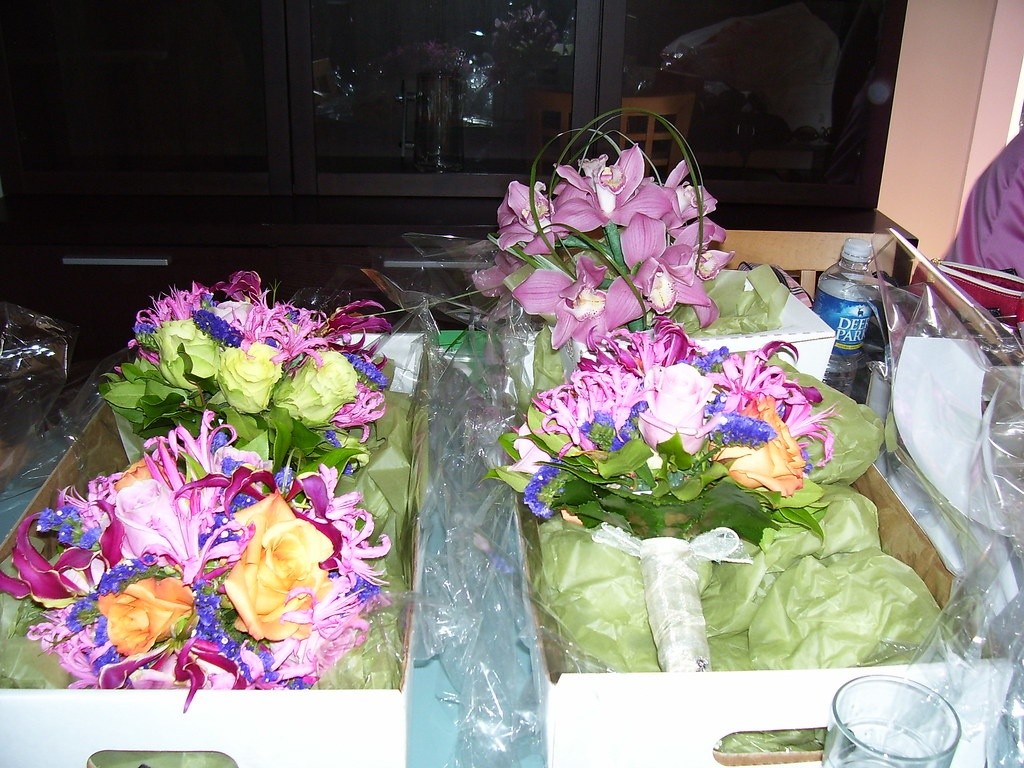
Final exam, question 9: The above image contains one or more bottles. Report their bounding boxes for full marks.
[812,237,873,398]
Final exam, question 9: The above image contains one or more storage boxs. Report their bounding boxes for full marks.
[0,332,1014,768]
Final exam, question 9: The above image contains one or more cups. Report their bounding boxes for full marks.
[821,674,961,768]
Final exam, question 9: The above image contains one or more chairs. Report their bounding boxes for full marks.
[708,230,897,300]
[532,91,696,180]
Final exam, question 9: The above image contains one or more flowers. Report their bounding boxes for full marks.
[384,40,469,75]
[0,107,882,713]
[492,3,558,55]
